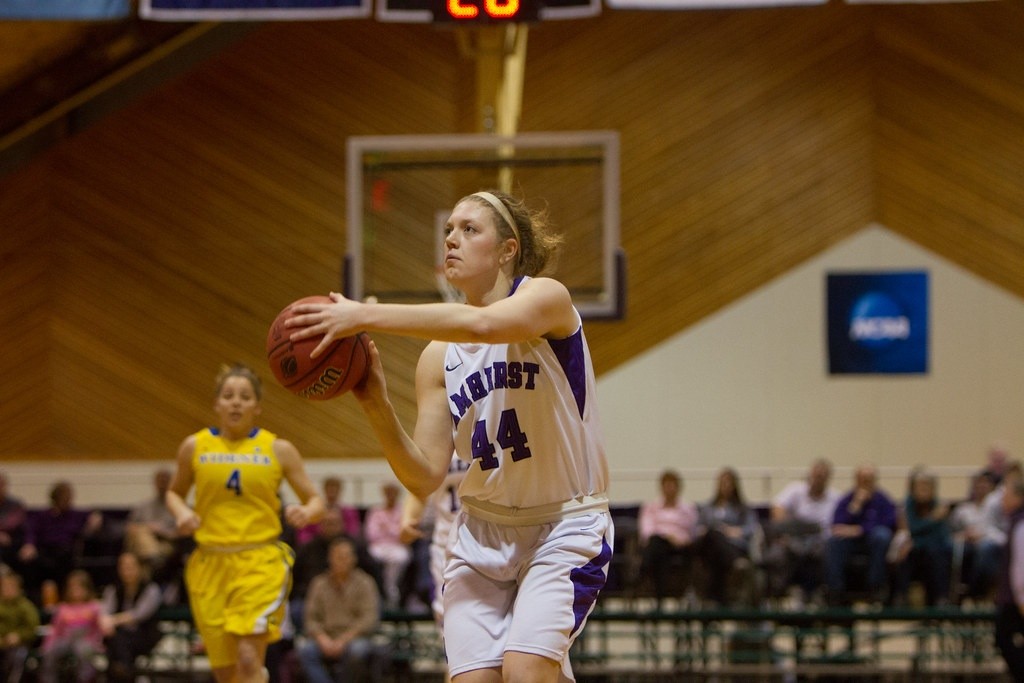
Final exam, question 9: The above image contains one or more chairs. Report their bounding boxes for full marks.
[0,505,1007,683]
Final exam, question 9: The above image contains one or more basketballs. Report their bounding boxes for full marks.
[265,288,379,402]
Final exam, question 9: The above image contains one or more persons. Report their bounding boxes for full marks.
[636,472,703,598]
[365,486,411,614]
[284,189,615,683]
[0,571,40,683]
[1,469,198,624]
[100,553,166,683]
[296,539,381,683]
[167,365,325,683]
[825,465,898,611]
[695,467,763,606]
[948,440,1024,683]
[297,476,361,549]
[769,458,842,610]
[894,466,956,609]
[41,570,104,683]
